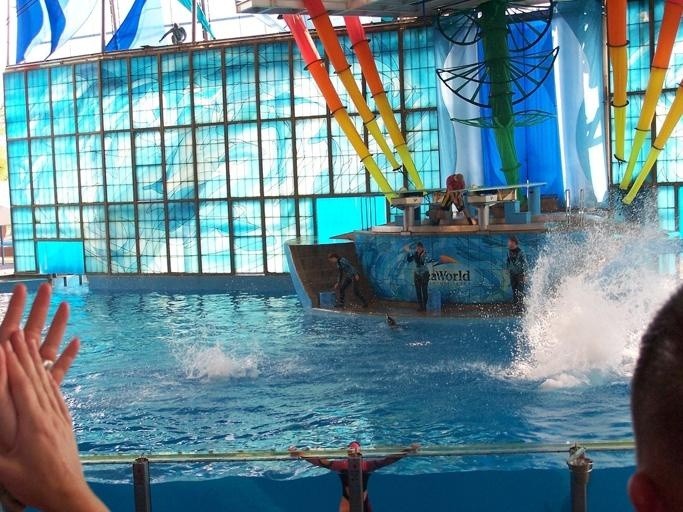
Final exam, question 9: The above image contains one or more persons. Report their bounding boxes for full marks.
[406,241,436,312]
[159,22,187,45]
[0,283,111,511]
[628,282,682,511]
[430,173,478,226]
[505,235,528,315]
[328,251,368,308]
[289,442,421,511]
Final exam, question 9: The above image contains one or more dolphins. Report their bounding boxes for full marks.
[385,313,399,330]
